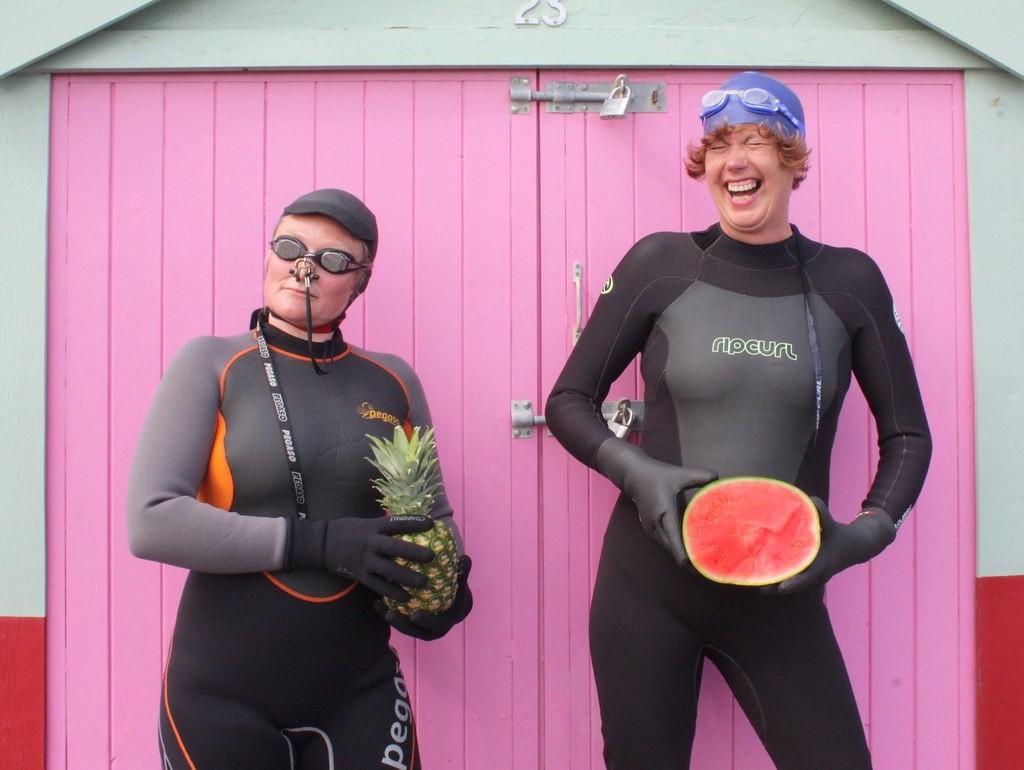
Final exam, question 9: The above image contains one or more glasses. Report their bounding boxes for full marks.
[271,236,374,275]
[698,87,780,119]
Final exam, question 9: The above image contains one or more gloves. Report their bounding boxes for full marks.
[594,436,721,567]
[761,496,896,596]
[376,555,473,640]
[283,513,435,604]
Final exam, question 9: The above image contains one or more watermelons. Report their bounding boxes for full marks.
[682,475,821,586]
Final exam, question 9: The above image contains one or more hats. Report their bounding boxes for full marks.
[285,188,378,249]
[703,72,806,144]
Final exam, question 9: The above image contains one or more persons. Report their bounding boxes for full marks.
[542,70,934,770]
[120,186,476,770]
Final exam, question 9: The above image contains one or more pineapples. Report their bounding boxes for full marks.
[362,426,459,614]
